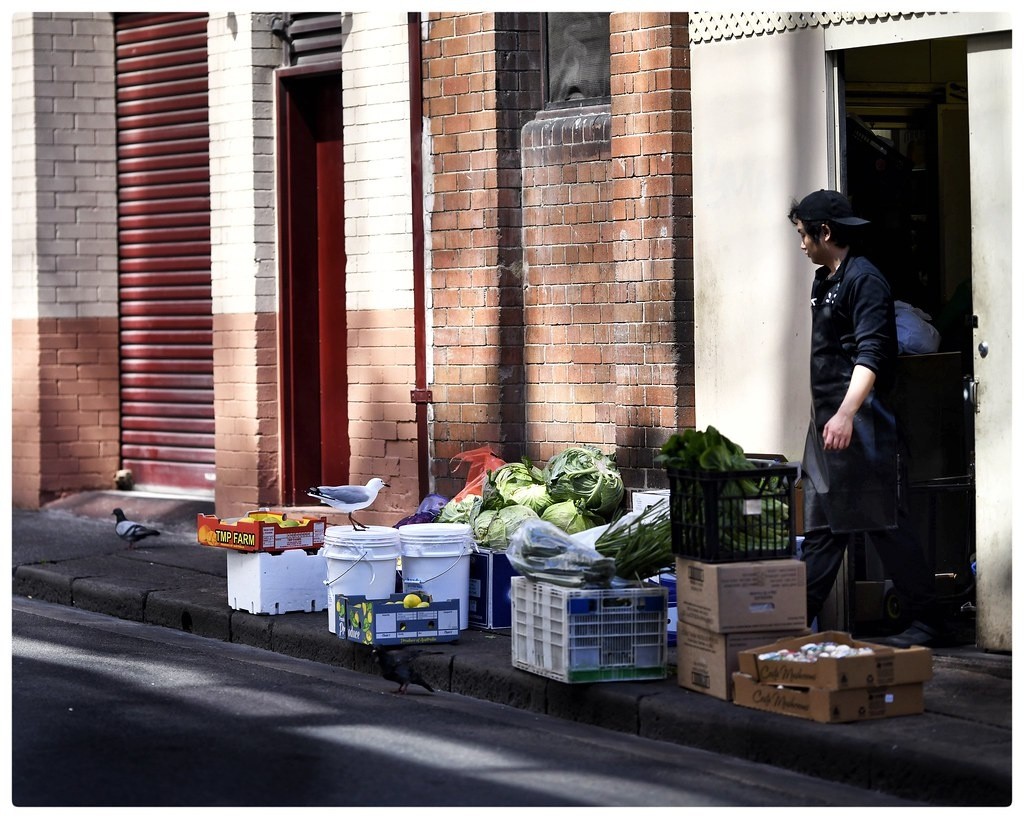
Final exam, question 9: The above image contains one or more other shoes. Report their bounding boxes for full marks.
[883,621,938,649]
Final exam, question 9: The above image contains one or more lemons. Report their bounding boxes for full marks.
[354,594,430,630]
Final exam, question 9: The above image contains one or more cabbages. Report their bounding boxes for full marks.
[433,447,624,550]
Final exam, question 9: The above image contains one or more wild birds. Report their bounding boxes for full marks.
[372,646,435,695]
[305,479,390,531]
[111,508,161,550]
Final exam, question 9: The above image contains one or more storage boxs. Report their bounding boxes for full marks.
[739,629,933,690]
[734,675,926,722]
[508,575,669,683]
[672,556,808,634]
[197,511,326,554]
[334,591,460,647]
[226,548,328,616]
[675,620,810,702]
[634,492,670,519]
[665,461,797,564]
[469,545,519,631]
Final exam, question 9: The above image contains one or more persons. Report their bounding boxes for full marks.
[787,189,961,651]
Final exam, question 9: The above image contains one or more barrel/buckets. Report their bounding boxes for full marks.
[322,525,401,633]
[399,523,473,630]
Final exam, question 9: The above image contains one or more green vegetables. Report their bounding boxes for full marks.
[652,425,789,554]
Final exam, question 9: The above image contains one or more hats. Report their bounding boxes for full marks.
[795,189,870,226]
